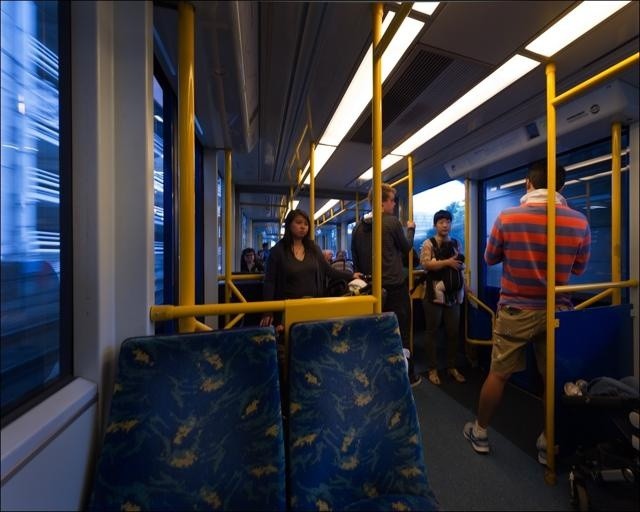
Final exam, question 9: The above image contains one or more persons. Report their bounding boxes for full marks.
[259,209,368,328]
[432,240,465,304]
[419,211,466,385]
[462,158,591,466]
[242,243,347,274]
[351,183,422,388]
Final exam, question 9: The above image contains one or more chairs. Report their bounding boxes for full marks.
[218,280,264,303]
[78,324,288,512]
[286,311,442,512]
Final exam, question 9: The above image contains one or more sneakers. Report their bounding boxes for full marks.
[536,433,548,465]
[429,371,439,384]
[411,375,422,387]
[449,369,465,382]
[463,423,489,453]
